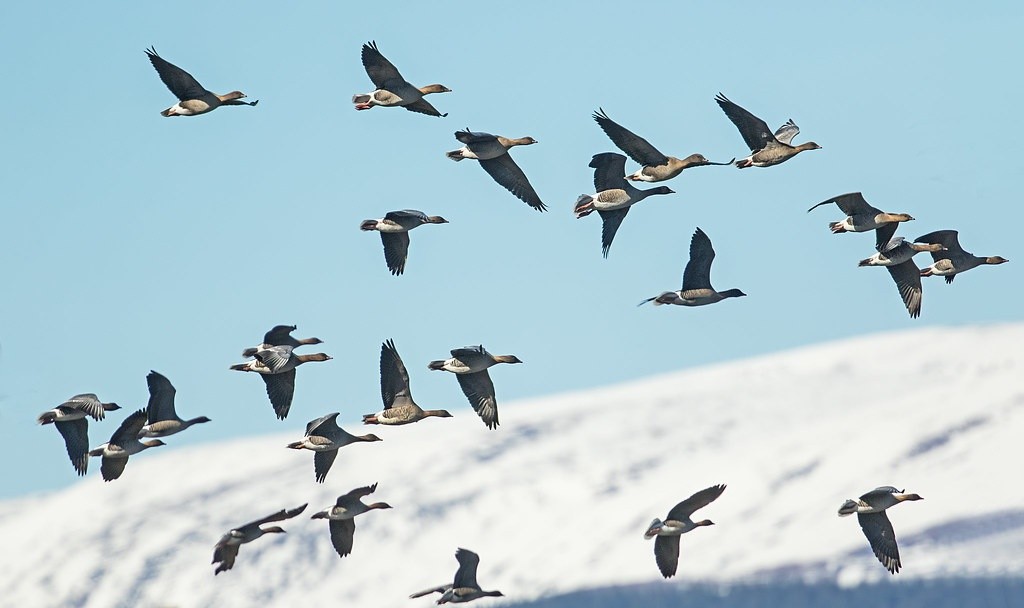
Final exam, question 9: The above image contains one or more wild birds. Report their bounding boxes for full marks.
[837,485,925,575]
[360,208,450,275]
[807,186,916,251]
[352,40,453,118]
[427,345,523,430]
[642,483,729,579]
[293,414,384,482]
[211,502,308,575]
[366,340,453,425]
[857,236,948,319]
[446,126,552,213]
[719,93,823,169]
[36,370,212,483]
[410,548,505,606]
[311,481,393,559]
[570,108,712,260]
[145,44,257,117]
[229,323,334,419]
[913,230,1010,285]
[634,226,747,306]
